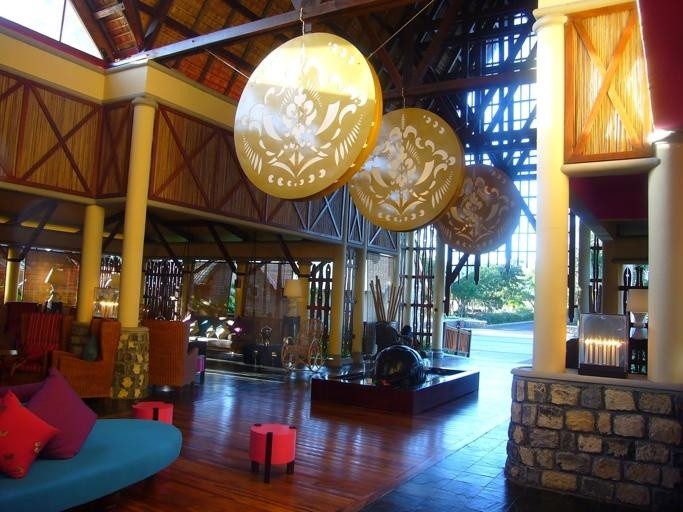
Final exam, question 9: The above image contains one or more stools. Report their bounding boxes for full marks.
[248,421,301,481]
[132,402,174,426]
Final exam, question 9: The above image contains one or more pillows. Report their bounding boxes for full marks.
[26,364,98,459]
[190,319,247,340]
[0,386,58,478]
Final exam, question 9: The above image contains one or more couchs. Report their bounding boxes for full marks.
[188,315,259,362]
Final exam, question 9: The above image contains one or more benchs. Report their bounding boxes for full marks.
[0,418,183,512]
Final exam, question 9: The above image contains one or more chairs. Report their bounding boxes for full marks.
[282,316,326,372]
[1,301,204,401]
